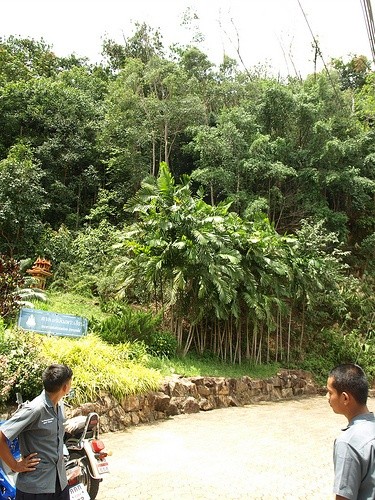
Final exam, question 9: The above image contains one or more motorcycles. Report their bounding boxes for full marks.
[1,412,110,499]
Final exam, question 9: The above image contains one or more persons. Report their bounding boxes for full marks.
[0,364,74,500]
[324,363,375,500]
